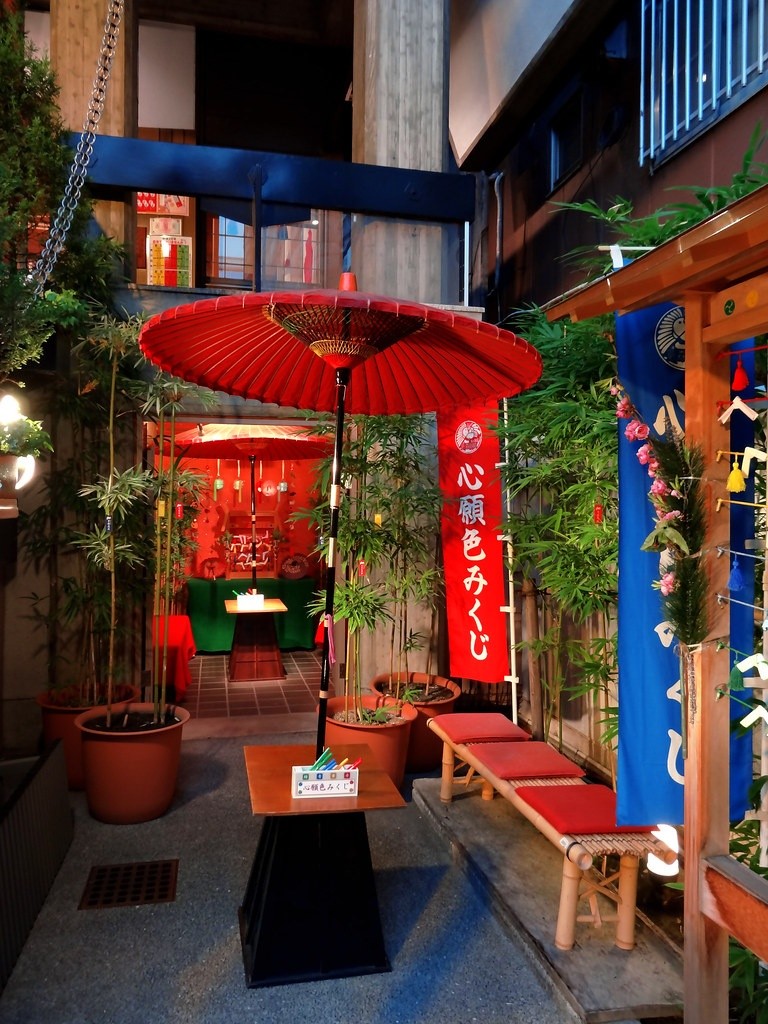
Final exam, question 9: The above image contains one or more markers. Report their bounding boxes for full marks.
[308,746,363,771]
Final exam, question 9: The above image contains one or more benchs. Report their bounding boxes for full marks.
[427,712,678,951]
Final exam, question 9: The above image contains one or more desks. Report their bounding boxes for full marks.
[237,744,408,988]
[153,615,196,704]
[185,577,317,654]
[224,598,290,682]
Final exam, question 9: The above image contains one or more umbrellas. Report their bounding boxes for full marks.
[151,422,341,598]
[139,273,544,767]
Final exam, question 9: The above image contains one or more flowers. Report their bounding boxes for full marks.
[611,382,682,596]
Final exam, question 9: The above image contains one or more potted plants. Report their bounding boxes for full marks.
[295,401,460,785]
[20,307,192,822]
